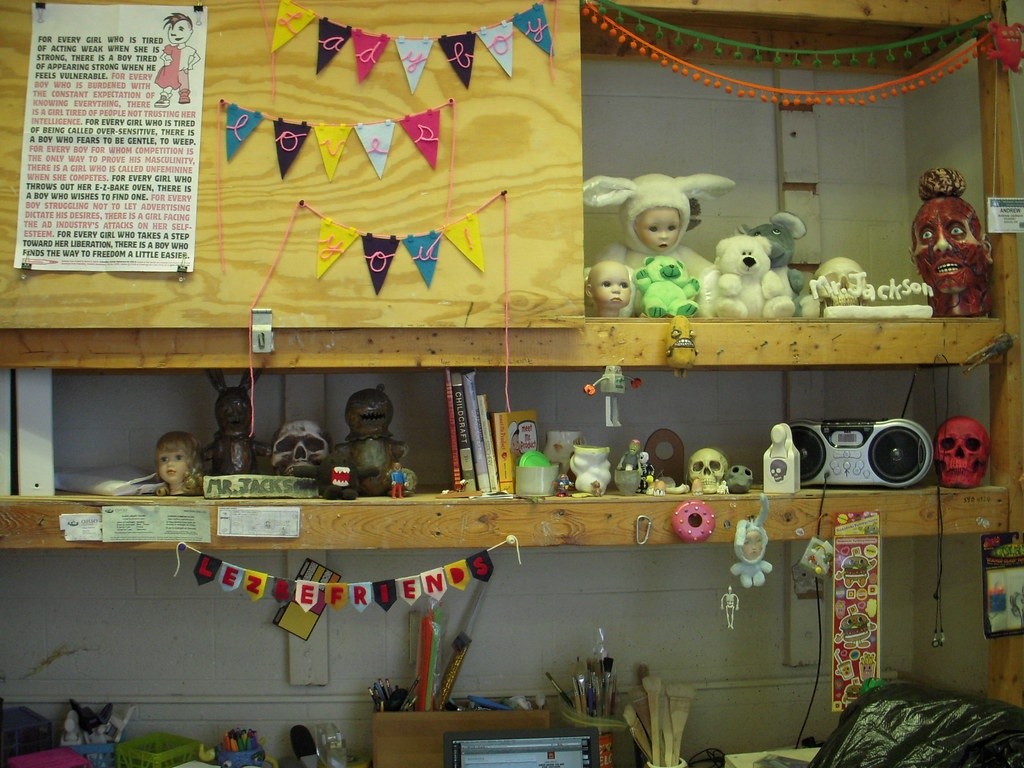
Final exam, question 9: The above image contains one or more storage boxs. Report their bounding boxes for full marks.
[371,697,550,768]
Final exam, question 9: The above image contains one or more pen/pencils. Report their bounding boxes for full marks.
[369,673,422,713]
[223,727,257,753]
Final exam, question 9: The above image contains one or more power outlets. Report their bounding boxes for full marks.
[407,609,446,664]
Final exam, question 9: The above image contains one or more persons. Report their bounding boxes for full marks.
[154,431,203,495]
[724,465,753,495]
[390,462,409,499]
[687,448,729,494]
[583,174,735,279]
[933,416,990,488]
[618,440,655,495]
[270,420,333,476]
[908,169,993,317]
[585,261,630,318]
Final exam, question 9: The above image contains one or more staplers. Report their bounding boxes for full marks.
[468,695,512,711]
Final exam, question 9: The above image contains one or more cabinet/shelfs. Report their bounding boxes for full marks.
[0,0,1024,708]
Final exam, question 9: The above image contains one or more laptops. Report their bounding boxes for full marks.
[443,725,600,768]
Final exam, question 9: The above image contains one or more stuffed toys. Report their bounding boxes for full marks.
[632,169,808,318]
[731,493,773,588]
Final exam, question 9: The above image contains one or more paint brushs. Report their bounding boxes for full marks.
[545,655,602,718]
[603,656,614,718]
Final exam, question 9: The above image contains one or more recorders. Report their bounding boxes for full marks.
[789,366,934,489]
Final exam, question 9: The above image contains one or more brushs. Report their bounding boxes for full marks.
[622,665,696,768]
